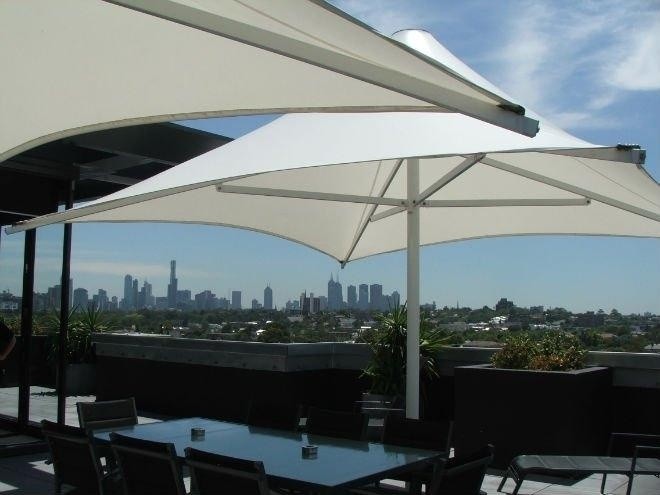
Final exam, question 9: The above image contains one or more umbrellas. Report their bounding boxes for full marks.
[0,0,540,165]
[5,29,660,421]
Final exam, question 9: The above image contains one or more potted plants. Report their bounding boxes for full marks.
[355,292,458,444]
[451,327,615,481]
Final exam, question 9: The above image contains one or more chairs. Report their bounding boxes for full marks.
[40,399,660,493]
